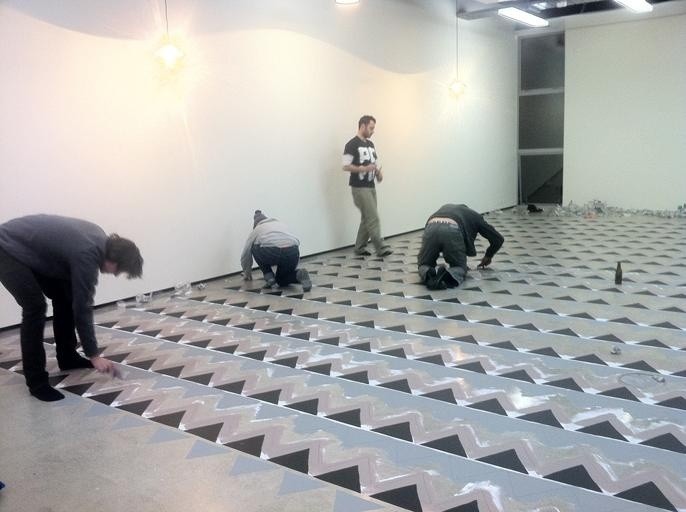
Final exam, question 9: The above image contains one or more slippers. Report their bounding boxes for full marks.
[300,269,312,292]
[262,283,282,294]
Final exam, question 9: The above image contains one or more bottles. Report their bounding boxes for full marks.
[615,262,624,284]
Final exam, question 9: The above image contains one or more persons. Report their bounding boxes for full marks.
[241,211,311,292]
[0,215,143,401]
[342,116,393,257]
[417,204,504,290]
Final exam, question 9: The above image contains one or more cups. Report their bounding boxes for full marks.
[116,302,126,315]
